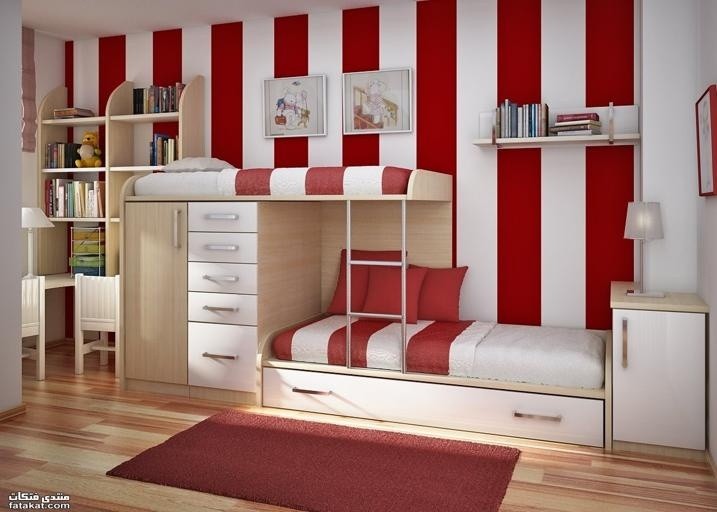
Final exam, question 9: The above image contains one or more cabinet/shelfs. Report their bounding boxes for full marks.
[36,73,207,291]
[119,196,186,399]
[610,280,707,456]
[186,198,323,399]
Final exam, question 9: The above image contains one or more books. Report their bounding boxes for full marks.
[52,108,97,118]
[132,82,184,173]
[39,137,107,223]
[485,95,605,139]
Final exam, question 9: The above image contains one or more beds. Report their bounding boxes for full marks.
[115,162,454,202]
[254,311,613,449]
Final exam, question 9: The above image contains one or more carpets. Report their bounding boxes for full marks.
[105,407,520,510]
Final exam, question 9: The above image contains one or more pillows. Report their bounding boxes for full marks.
[361,263,426,323]
[327,249,408,315]
[160,155,237,172]
[406,265,469,324]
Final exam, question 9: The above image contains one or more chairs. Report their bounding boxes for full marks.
[73,273,118,378]
[21,276,46,383]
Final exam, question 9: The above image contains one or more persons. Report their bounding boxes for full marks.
[74,131,103,168]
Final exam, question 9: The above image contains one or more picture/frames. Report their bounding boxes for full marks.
[262,74,327,140]
[341,67,414,137]
[694,82,717,196]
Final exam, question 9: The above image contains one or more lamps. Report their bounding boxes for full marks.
[623,199,666,299]
[21,207,55,280]
